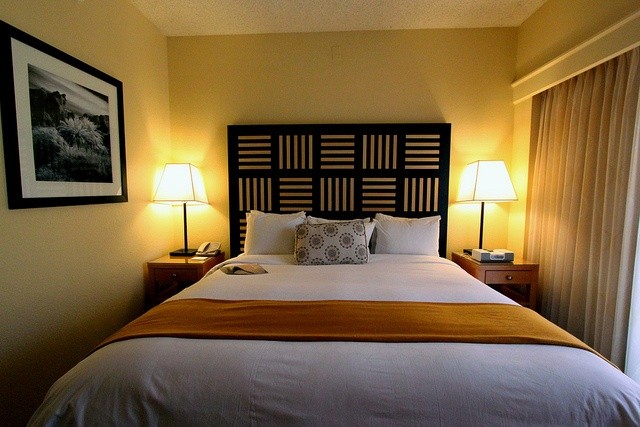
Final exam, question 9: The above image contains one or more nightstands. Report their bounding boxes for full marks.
[451,251,540,312]
[146,251,225,310]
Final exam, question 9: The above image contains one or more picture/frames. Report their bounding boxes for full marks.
[0,20,129,209]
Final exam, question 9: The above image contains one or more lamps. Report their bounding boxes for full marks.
[153,162,210,256]
[455,159,518,255]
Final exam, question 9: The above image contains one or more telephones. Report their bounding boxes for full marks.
[195,242,222,256]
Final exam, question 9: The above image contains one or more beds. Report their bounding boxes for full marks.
[31,209,640,427]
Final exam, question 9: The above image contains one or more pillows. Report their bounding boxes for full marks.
[374,212,441,257]
[294,220,369,265]
[307,215,376,249]
[244,210,307,255]
